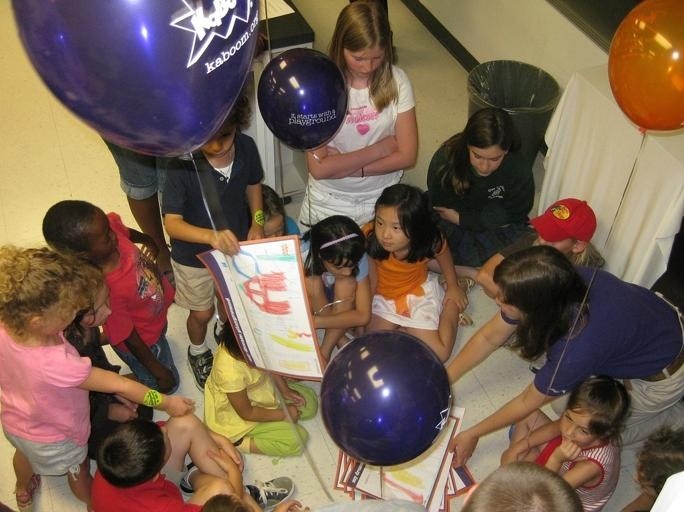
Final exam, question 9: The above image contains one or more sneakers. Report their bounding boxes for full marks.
[186,344,217,390]
[244,477,296,510]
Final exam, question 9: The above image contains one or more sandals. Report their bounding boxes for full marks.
[13,472,40,511]
[440,273,477,327]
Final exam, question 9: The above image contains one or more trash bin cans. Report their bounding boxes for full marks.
[466,59,561,168]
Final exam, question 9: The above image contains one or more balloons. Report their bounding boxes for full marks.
[9,0,261,161]
[608,0,684,132]
[317,330,450,465]
[257,48,349,152]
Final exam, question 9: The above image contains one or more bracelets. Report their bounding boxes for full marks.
[361,167,364,176]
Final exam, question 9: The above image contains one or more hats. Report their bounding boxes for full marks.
[530,197,598,245]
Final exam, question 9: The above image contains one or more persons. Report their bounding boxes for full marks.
[443,245,684,466]
[259,181,300,237]
[424,107,534,268]
[42,200,180,395]
[361,183,474,363]
[0,244,196,511]
[161,79,265,393]
[295,215,371,371]
[475,198,604,373]
[90,412,243,512]
[460,460,586,511]
[617,423,684,511]
[500,376,632,512]
[60,258,153,461]
[200,493,313,512]
[101,138,176,303]
[202,315,318,457]
